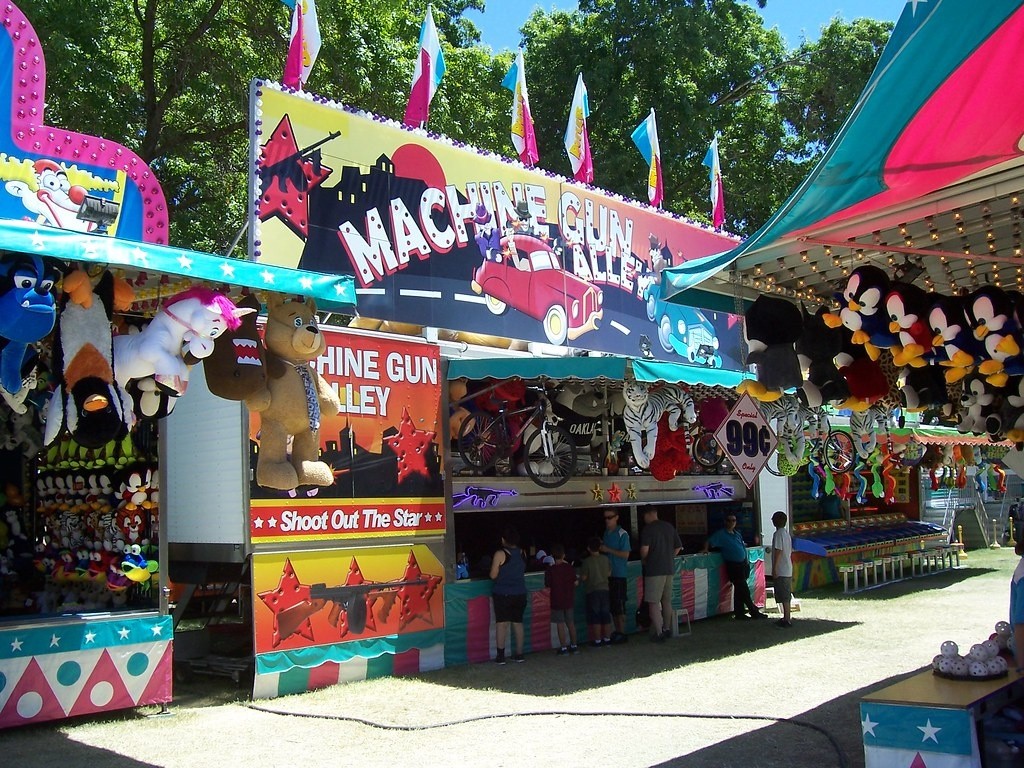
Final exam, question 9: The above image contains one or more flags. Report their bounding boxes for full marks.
[402,11,447,129]
[629,113,664,207]
[563,78,594,184]
[701,138,724,228]
[279,0,323,93]
[499,55,539,166]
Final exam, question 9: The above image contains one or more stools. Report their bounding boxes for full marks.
[669,609,691,638]
[765,587,801,613]
[836,542,964,595]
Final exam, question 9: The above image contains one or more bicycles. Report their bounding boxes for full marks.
[457,384,579,489]
[763,411,857,477]
[686,409,726,469]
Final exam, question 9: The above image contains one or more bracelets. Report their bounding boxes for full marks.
[1015,668,1024,673]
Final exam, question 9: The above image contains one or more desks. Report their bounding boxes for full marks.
[860,666,1022,768]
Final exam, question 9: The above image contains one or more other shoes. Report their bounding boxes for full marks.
[662,627,671,638]
[778,621,792,627]
[751,613,768,618]
[775,618,785,623]
[559,648,568,655]
[651,632,664,642]
[569,645,577,653]
[602,638,611,645]
[494,658,505,665]
[612,632,617,641]
[589,640,602,647]
[510,656,525,662]
[614,633,628,643]
[735,615,751,621]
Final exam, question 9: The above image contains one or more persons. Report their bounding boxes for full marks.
[488,528,528,665]
[544,504,683,653]
[700,510,794,631]
[1010,559,1024,676]
[1008,497,1024,541]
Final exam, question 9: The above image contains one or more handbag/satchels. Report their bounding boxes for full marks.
[636,608,651,628]
[734,560,751,581]
[565,567,576,591]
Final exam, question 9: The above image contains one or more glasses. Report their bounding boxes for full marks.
[726,519,736,522]
[604,514,617,520]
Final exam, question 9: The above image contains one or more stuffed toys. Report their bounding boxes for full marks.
[201,291,342,490]
[0,396,161,621]
[43,266,136,451]
[776,435,1009,503]
[736,259,1024,466]
[444,371,730,484]
[0,252,60,394]
[112,280,258,434]
